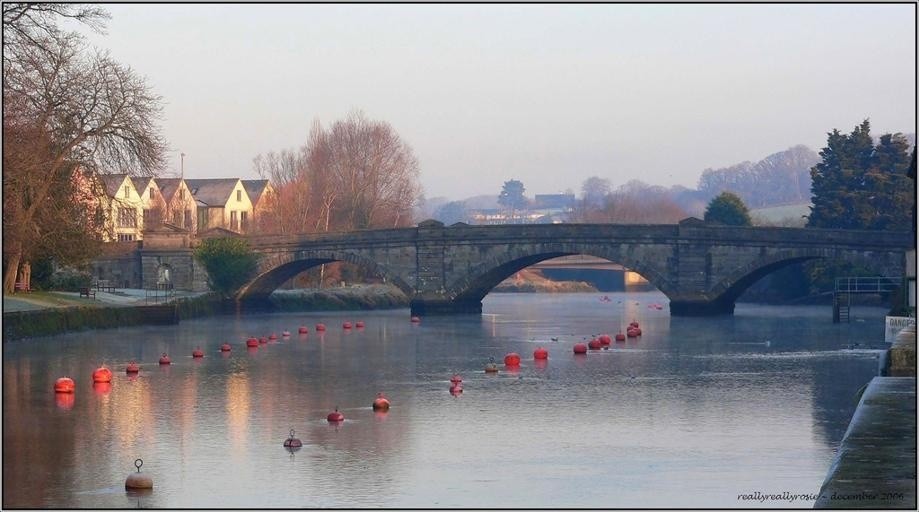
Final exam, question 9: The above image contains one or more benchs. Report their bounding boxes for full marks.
[79,280,115,301]
